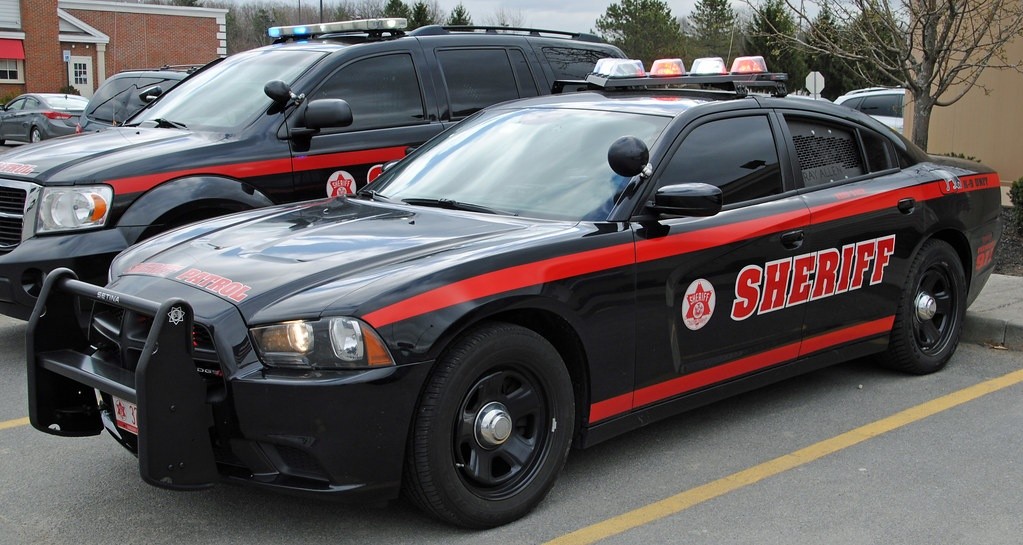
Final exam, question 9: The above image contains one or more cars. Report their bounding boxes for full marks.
[832,86,907,137]
[75,62,209,138]
[22,58,1004,530]
[0,92,91,145]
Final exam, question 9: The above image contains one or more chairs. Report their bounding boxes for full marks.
[340,50,519,121]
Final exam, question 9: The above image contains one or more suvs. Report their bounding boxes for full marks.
[0,17,650,324]
